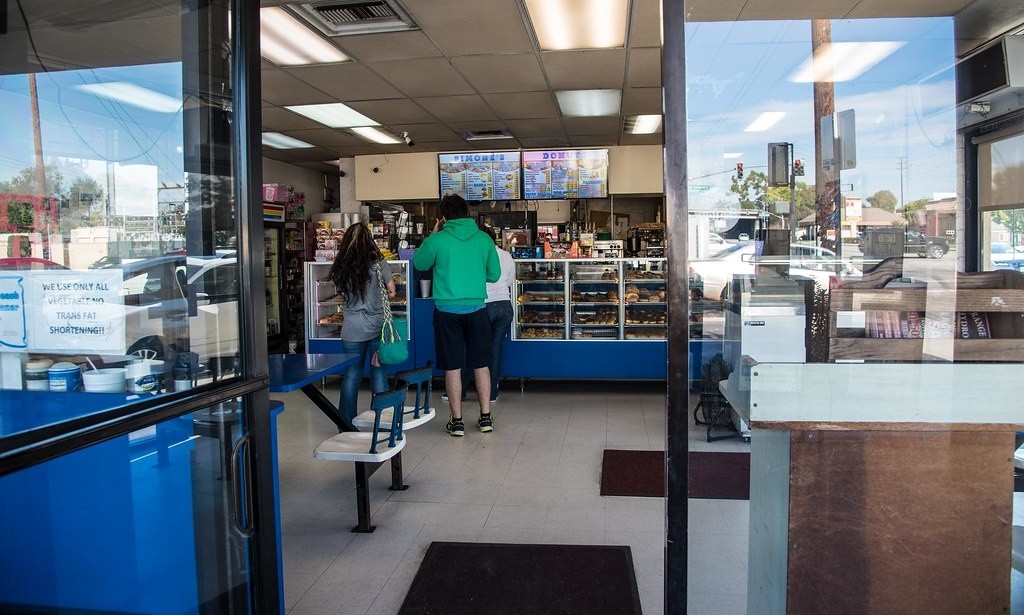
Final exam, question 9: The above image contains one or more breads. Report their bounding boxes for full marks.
[320,274,407,323]
[515,270,666,339]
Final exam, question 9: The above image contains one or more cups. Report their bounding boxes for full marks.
[416,223,424,234]
[420,280,431,297]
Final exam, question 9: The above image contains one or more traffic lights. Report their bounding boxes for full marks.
[736,162,744,179]
[792,159,804,177]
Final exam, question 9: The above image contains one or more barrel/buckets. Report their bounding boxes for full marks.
[82,367,127,393]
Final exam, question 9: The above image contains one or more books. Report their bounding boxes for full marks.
[864,310,991,340]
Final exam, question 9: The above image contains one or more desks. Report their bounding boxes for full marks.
[265,351,361,535]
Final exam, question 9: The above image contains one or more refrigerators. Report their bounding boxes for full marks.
[231,198,287,351]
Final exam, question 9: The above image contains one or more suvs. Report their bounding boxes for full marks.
[857,228,950,260]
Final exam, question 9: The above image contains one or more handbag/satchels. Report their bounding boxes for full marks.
[377,318,409,364]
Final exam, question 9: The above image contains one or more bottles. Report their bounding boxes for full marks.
[265,246,276,334]
[566,221,597,241]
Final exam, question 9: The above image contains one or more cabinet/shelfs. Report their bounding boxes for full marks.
[304,260,721,385]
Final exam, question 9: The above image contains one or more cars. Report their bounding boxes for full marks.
[684,231,874,306]
[87,247,240,389]
[991,241,1024,273]
[0,255,76,280]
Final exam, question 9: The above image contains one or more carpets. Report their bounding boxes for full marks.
[398,540,644,615]
[600,449,751,499]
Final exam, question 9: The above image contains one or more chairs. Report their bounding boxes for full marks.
[351,360,436,491]
[313,387,406,534]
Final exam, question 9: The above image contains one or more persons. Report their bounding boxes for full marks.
[328,222,395,432]
[413,193,502,437]
[441,226,516,403]
[858,190,903,282]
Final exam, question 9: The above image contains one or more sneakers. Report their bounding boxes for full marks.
[445,414,465,436]
[476,415,494,432]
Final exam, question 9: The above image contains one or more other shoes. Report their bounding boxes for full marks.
[442,391,466,401]
[490,391,501,402]
